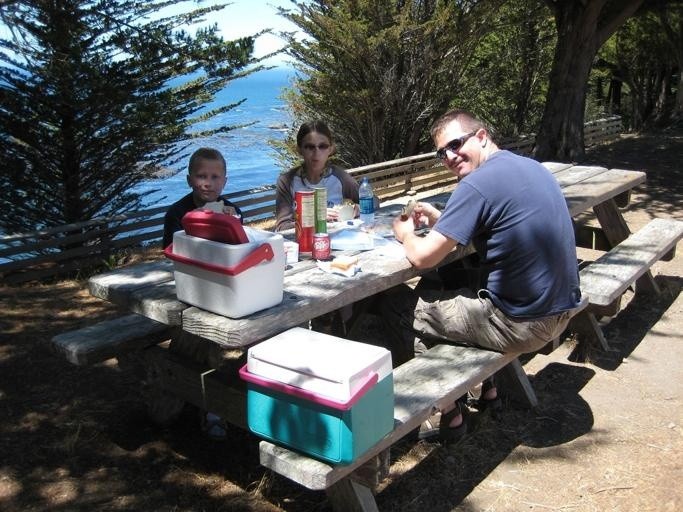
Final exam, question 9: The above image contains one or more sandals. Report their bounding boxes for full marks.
[202,408,229,441]
[412,381,501,438]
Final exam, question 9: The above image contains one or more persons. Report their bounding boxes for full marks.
[275,119,360,231]
[392,109,581,438]
[163,148,243,252]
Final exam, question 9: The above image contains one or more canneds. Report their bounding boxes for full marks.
[313,232,331,260]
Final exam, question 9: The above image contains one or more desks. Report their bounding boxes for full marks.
[79,150,652,510]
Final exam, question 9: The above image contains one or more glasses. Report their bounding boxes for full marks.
[436,132,476,159]
[298,143,331,152]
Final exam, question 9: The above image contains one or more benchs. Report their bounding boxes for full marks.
[252,292,594,511]
[577,214,683,353]
[47,314,183,442]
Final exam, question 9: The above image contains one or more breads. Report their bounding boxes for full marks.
[330,254,359,269]
[401,198,421,221]
[333,203,356,222]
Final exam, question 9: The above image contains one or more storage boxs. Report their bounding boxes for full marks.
[238,322,395,466]
[161,210,286,320]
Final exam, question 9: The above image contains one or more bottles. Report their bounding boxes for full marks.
[358,177,374,229]
